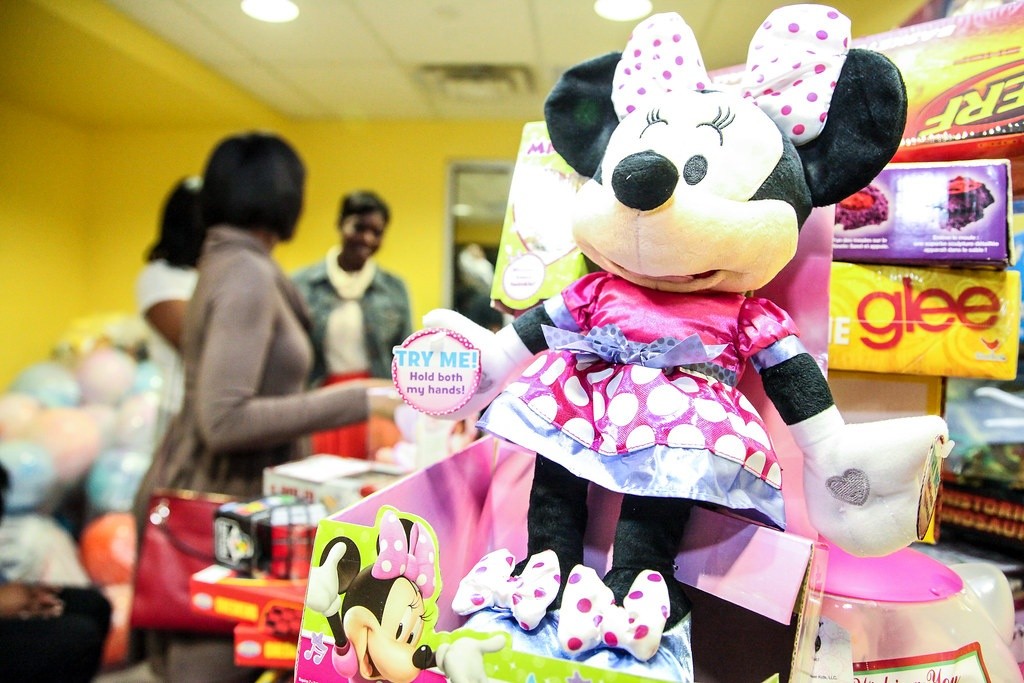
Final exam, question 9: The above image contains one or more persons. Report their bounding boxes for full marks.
[286,192,413,459]
[448,297,503,449]
[143,175,205,443]
[0,462,114,683]
[121,133,388,682]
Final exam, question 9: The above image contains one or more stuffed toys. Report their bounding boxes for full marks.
[391,5,948,682]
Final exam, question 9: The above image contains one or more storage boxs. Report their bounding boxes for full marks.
[489,121,600,317]
[233,623,298,671]
[827,259,1021,383]
[827,368,948,546]
[705,0,1024,201]
[263,452,414,514]
[938,471,1024,567]
[189,563,308,640]
[293,435,832,683]
[833,156,1018,273]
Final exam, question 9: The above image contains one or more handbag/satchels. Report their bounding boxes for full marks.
[131,487,239,635]
